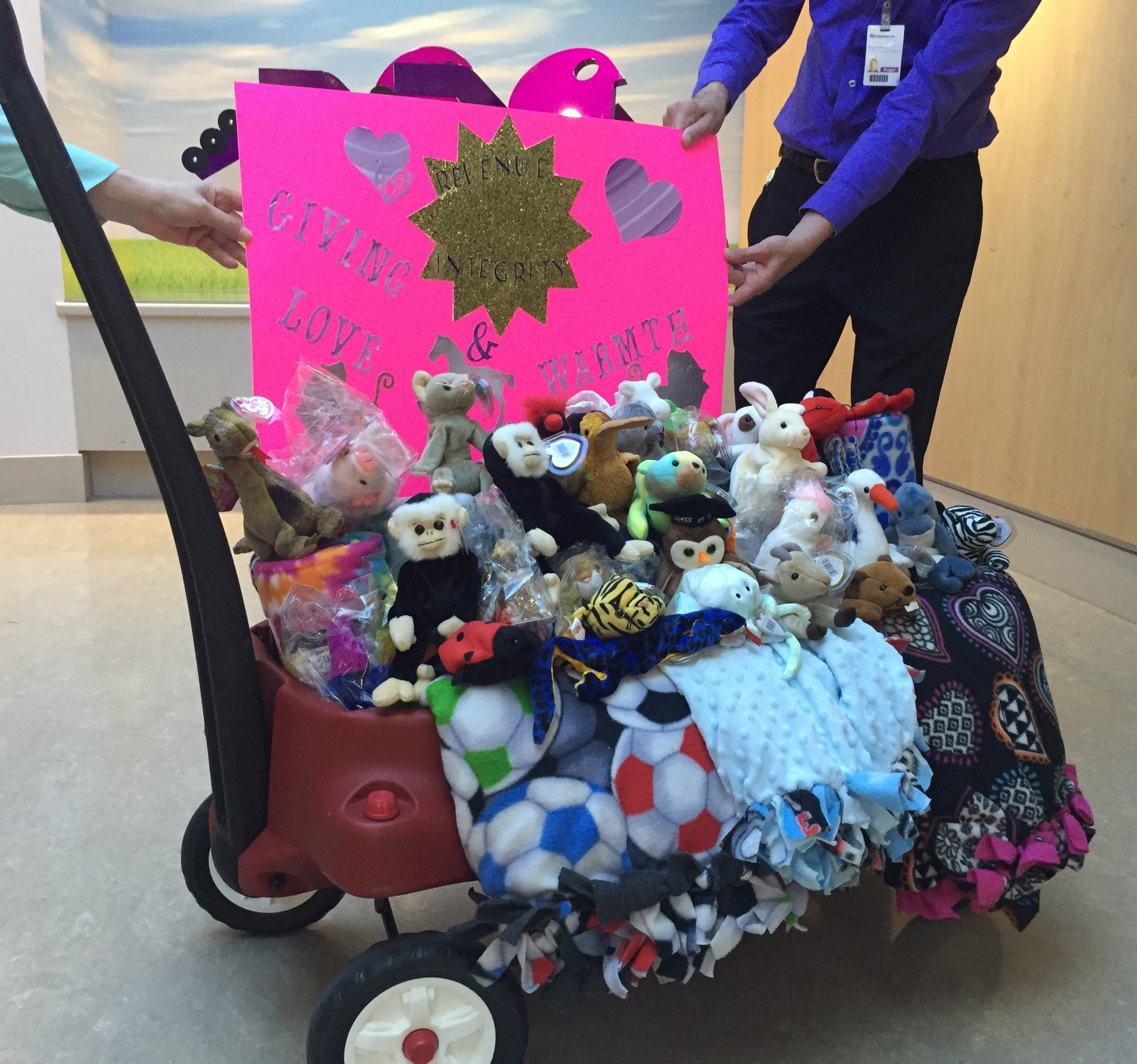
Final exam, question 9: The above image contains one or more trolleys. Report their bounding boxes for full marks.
[1,2,937,1064]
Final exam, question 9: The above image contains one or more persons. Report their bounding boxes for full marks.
[0,103,252,270]
[659,1,1043,485]
[867,58,879,73]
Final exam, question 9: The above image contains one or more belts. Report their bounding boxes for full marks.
[780,146,836,184]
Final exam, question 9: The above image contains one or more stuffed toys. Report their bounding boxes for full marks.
[186,370,1011,749]
